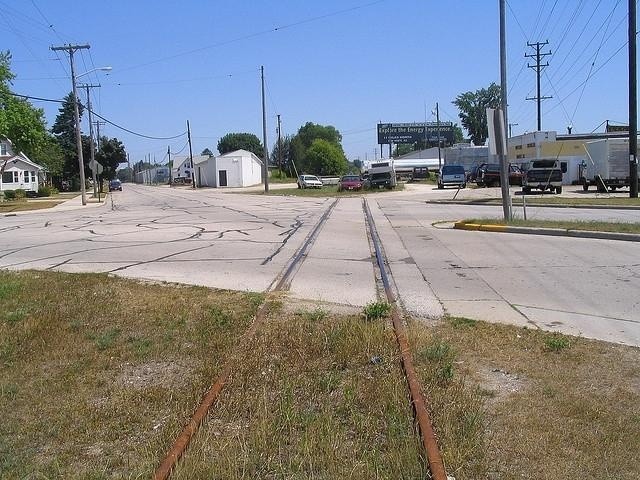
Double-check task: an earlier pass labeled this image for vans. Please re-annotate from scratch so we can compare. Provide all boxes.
[520,157,563,193]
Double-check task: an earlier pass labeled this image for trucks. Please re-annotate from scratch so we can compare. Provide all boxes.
[581,134,640,193]
[359,158,444,180]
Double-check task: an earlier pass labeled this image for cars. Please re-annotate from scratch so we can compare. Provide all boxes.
[295,174,324,190]
[109,178,123,192]
[335,174,362,193]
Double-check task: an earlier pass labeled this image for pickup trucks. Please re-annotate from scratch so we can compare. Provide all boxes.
[412,166,430,182]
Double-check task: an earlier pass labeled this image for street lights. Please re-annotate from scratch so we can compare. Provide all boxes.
[73,67,112,206]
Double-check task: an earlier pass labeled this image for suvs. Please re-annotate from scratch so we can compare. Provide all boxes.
[475,161,524,188]
[371,172,394,190]
[436,163,467,190]
